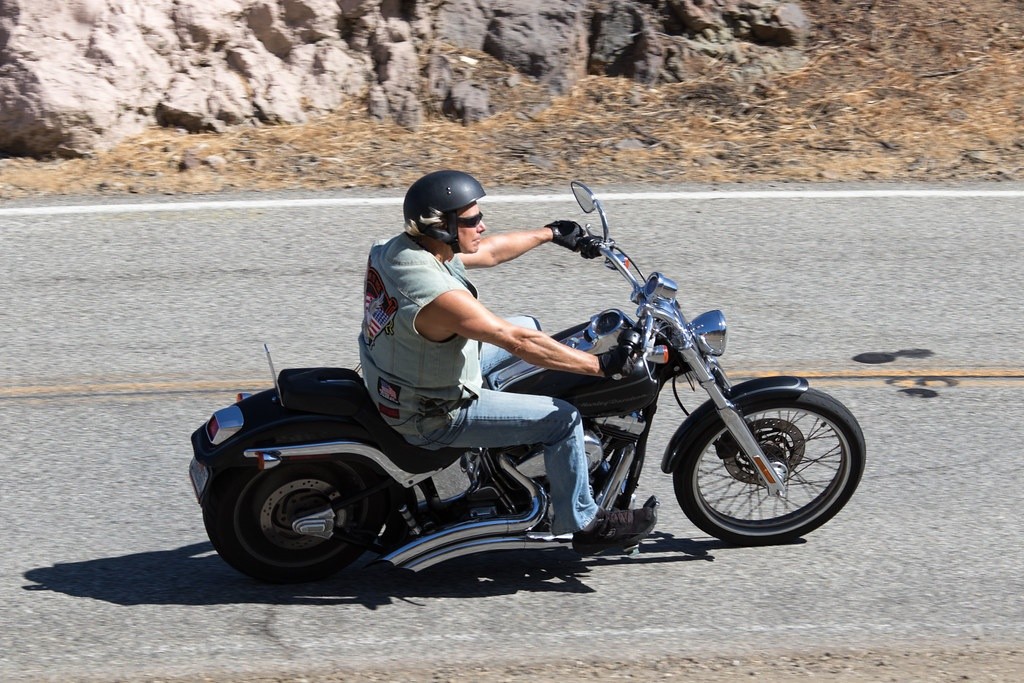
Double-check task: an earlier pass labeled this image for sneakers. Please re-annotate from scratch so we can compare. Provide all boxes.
[572,507,657,556]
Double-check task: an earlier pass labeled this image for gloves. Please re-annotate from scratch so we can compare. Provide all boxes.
[600,348,637,379]
[545,219,585,253]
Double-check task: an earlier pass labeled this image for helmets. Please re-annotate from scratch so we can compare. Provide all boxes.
[404,170,485,226]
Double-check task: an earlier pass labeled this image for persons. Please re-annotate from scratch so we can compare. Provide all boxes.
[359,170,658,555]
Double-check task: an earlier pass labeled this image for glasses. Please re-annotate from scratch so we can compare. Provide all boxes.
[455,212,483,228]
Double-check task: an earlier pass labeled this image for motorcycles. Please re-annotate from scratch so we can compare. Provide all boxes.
[188,177,870,587]
